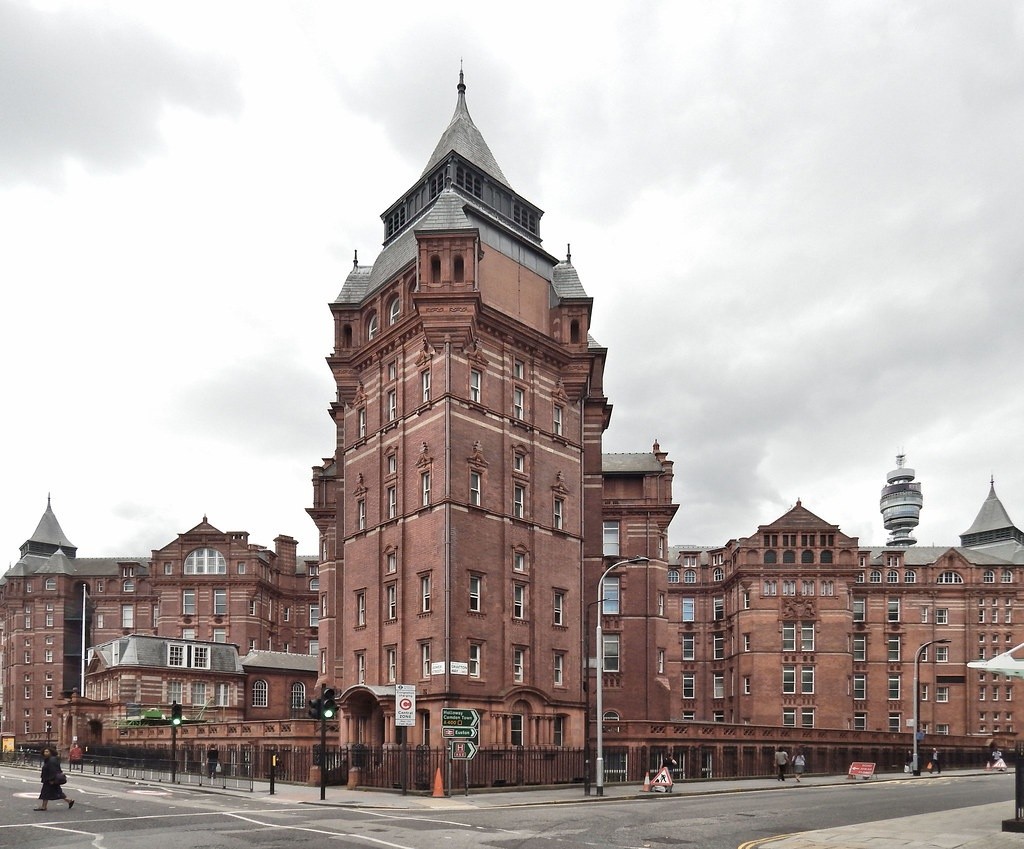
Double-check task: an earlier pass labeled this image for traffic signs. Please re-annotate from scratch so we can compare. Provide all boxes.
[441,708,480,760]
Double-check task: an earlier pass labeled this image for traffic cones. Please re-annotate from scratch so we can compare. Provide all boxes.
[639,772,651,792]
[984,761,992,771]
[430,769,451,799]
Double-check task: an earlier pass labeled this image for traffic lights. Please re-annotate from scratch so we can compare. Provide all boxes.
[172,704,183,727]
[308,698,322,719]
[323,688,337,719]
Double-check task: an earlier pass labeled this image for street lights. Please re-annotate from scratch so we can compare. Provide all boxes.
[594,558,652,795]
[583,598,611,796]
[912,639,952,776]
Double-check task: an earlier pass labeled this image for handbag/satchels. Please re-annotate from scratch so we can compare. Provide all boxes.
[927,762,932,769]
[672,759,677,765]
[791,755,797,767]
[53,770,67,785]
[904,765,909,772]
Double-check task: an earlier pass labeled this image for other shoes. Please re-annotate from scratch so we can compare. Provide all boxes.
[777,777,780,781]
[797,779,800,782]
[781,778,785,781]
[33,807,47,811]
[69,800,74,808]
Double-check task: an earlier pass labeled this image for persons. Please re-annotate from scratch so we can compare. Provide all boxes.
[662,753,676,793]
[791,748,805,782]
[33,747,75,812]
[992,748,1002,761]
[68,743,82,769]
[929,747,941,774]
[904,749,913,774]
[774,746,790,782]
